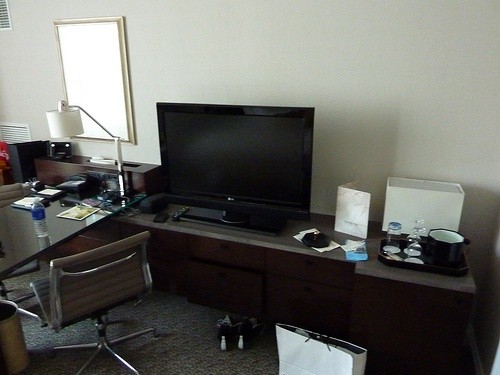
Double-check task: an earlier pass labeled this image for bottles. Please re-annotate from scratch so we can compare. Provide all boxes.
[31,197,49,238]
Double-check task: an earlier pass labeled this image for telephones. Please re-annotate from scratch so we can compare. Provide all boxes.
[55,175,100,195]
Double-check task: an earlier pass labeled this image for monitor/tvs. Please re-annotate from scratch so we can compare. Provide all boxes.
[156,102,315,235]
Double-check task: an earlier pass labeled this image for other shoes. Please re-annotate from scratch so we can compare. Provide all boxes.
[216,314,264,350]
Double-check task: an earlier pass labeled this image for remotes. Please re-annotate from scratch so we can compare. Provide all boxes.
[170,206,190,221]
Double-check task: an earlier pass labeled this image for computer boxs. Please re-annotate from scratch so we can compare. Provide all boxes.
[6,140,51,183]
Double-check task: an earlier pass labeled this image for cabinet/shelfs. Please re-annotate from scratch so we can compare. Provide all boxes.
[31,153,478,375]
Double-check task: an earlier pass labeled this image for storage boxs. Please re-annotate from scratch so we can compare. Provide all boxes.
[382,176,464,237]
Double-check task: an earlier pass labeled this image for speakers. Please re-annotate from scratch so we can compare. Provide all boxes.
[51,142,72,159]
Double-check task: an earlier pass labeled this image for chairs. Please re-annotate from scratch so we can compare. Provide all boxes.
[0,183,48,328]
[31,230,161,375]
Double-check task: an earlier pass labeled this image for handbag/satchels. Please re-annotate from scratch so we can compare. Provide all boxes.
[274,322,368,375]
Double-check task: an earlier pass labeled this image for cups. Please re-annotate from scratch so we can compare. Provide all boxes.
[387,221,402,253]
[428,228,465,267]
[354,239,367,254]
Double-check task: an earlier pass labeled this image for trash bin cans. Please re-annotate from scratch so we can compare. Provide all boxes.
[0,300,31,375]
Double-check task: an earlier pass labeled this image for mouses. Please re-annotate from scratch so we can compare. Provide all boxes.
[154,212,168,222]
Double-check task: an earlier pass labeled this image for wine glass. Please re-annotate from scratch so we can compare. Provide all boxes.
[406,217,428,257]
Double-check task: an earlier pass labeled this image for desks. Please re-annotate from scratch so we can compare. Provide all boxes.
[0,187,139,276]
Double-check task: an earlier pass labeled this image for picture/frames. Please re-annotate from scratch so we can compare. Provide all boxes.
[53,16,137,146]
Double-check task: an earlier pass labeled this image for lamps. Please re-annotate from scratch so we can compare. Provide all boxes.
[46,100,128,197]
